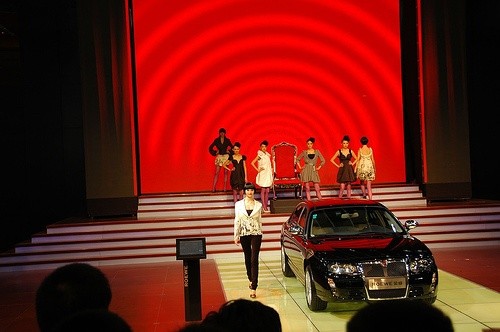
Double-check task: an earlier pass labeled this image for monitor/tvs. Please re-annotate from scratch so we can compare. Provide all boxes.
[176,238,206,260]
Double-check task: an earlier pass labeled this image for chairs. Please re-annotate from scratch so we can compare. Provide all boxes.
[272,142,303,201]
[314,210,372,233]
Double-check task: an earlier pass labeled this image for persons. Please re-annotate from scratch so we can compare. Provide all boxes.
[347,300,456,332]
[330,136,358,199]
[233,183,263,298]
[33,263,113,332]
[208,128,233,193]
[68,308,133,332]
[223,142,247,207]
[354,137,376,200]
[203,299,282,332]
[295,136,326,201]
[251,140,274,211]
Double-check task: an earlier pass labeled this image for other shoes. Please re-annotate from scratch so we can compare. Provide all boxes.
[249,284,252,289]
[250,294,256,298]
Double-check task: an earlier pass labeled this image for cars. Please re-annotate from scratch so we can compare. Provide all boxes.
[281,197,439,312]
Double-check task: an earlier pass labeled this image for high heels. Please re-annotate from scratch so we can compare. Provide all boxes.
[262,208,271,213]
